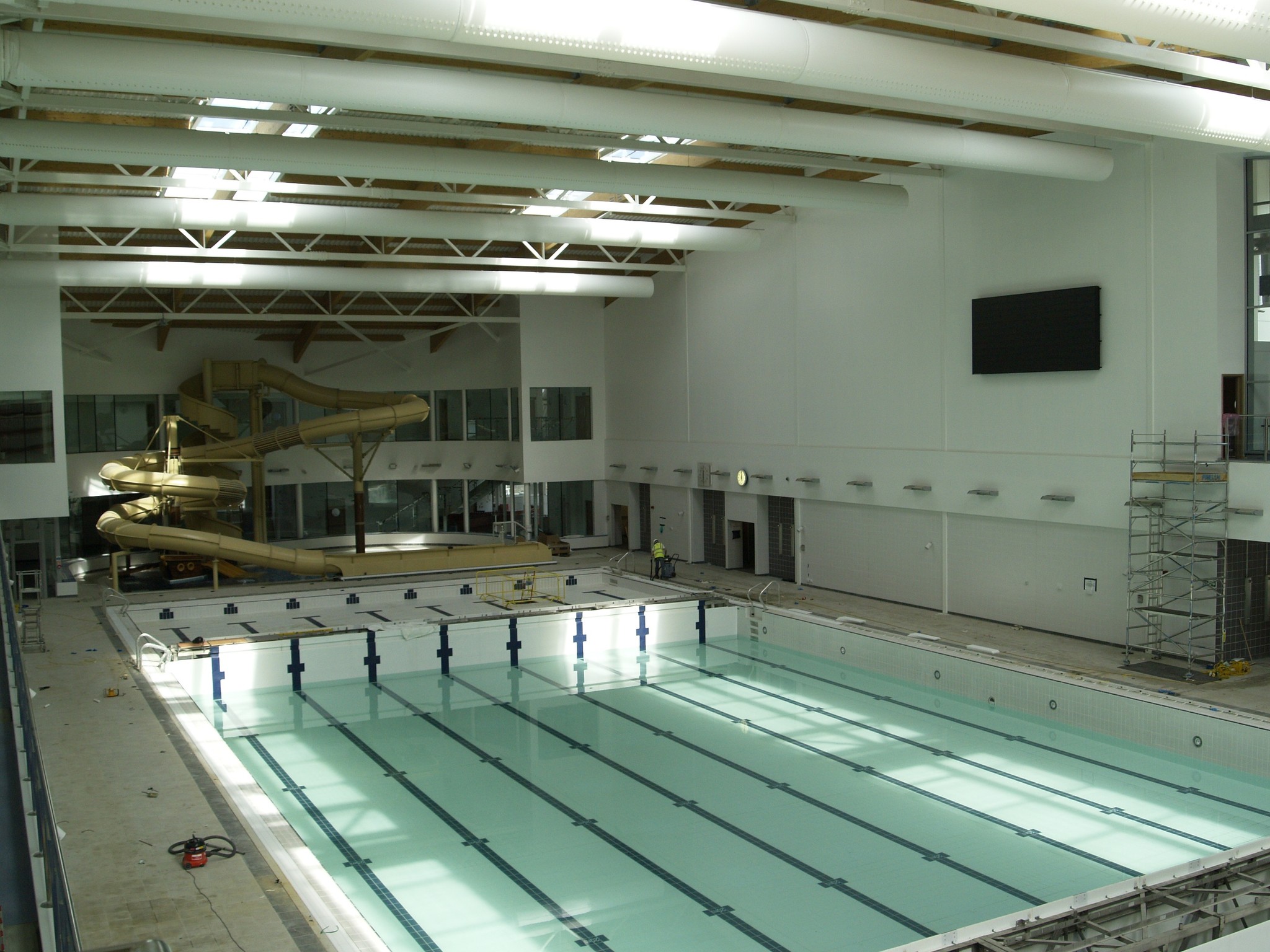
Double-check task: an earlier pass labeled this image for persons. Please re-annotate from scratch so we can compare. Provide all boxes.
[652,539,667,580]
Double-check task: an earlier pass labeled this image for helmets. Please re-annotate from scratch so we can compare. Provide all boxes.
[653,539,658,544]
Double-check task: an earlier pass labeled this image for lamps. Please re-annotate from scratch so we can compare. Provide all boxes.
[847,481,872,488]
[609,463,626,468]
[750,473,773,480]
[389,463,398,469]
[794,477,819,484]
[510,189,594,216]
[598,134,697,164]
[1124,501,1163,508]
[639,466,657,471]
[463,463,471,468]
[967,490,999,497]
[1041,495,1075,502]
[158,97,274,199]
[710,470,730,477]
[233,108,341,201]
[903,485,931,491]
[672,468,692,473]
[1222,508,1264,516]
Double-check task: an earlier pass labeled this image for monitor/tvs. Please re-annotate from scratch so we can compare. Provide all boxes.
[972,285,1101,375]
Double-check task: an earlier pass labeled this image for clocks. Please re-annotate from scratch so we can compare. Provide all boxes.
[737,470,747,486]
